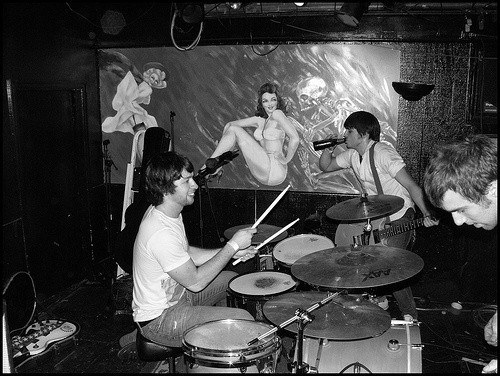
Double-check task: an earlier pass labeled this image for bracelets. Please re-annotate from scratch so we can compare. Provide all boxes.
[227,240,239,252]
[325,148,333,154]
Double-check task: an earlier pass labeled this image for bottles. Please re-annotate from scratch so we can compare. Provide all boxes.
[313,138,346,151]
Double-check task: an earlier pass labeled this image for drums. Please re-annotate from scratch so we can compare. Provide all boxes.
[182,318,281,373]
[226,269,296,326]
[273,234,335,273]
[292,319,424,373]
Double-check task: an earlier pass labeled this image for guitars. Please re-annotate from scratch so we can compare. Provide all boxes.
[11,320,76,358]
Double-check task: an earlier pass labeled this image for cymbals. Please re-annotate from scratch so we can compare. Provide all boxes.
[291,245,424,289]
[224,224,288,243]
[263,291,391,340]
[326,195,404,220]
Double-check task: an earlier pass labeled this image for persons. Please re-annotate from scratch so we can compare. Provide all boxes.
[132,152,258,347]
[319,112,439,320]
[425,134,497,373]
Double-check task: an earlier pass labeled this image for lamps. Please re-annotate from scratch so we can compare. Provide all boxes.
[335,2,372,28]
[170,2,203,34]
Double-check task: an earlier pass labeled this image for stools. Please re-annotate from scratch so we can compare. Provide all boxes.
[135,328,180,373]
